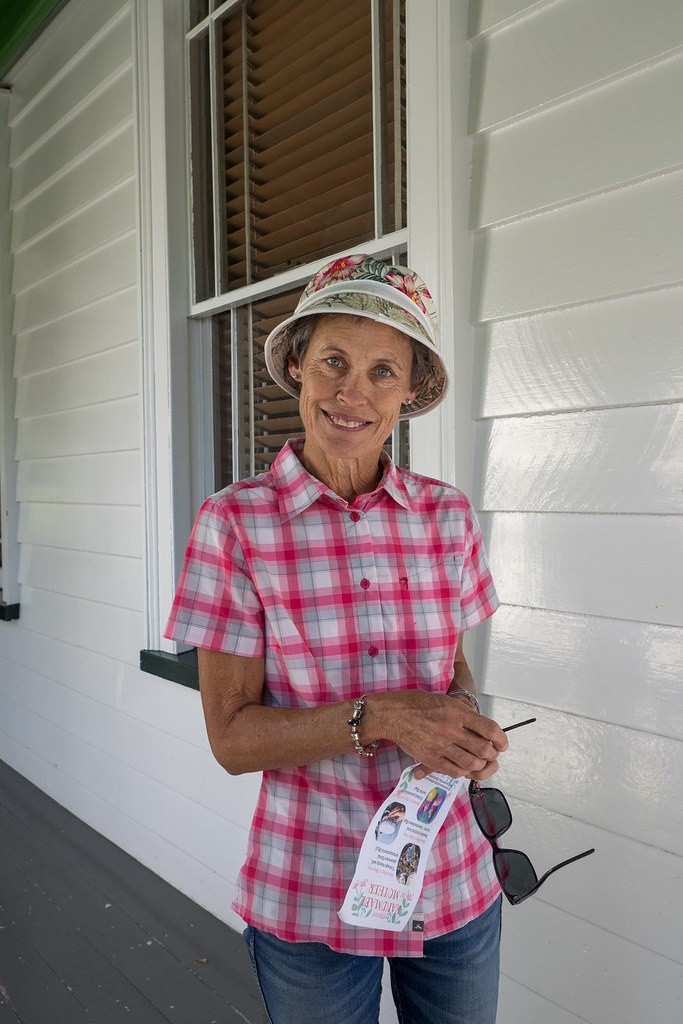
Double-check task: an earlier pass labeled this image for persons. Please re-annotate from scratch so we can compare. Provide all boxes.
[161,256,507,1024]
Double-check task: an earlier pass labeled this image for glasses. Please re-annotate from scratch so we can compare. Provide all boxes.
[465,718,596,907]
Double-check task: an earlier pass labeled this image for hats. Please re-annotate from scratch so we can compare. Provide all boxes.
[263,253,449,424]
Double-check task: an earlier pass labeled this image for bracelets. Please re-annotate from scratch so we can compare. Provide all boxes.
[447,689,482,716]
[346,693,379,758]
